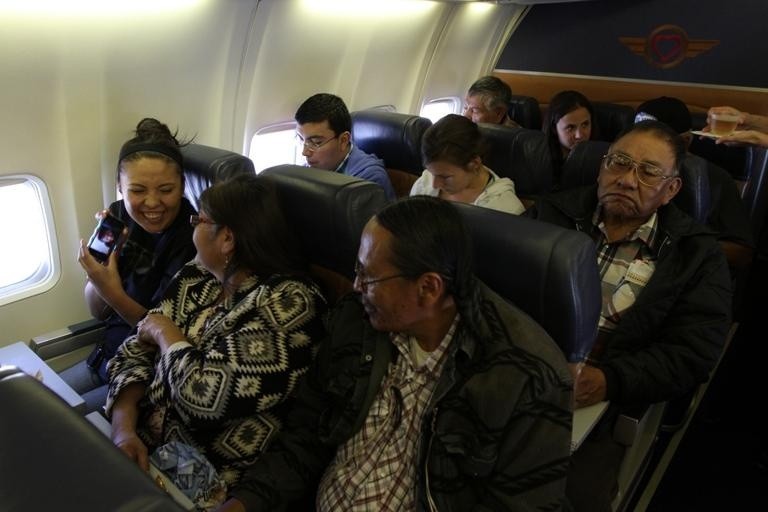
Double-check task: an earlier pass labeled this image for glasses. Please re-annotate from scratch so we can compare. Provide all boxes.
[355,256,453,293]
[190,215,216,227]
[295,134,335,152]
[601,151,681,187]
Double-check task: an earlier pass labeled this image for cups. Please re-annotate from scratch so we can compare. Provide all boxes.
[710,107,740,135]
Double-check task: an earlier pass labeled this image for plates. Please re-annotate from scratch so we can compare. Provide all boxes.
[690,128,741,140]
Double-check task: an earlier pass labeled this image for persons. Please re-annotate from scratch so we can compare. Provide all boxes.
[513,121,735,510]
[460,74,523,132]
[103,173,329,510]
[542,89,599,188]
[635,96,767,295]
[203,194,576,510]
[55,115,201,418]
[294,92,399,209]
[406,113,528,215]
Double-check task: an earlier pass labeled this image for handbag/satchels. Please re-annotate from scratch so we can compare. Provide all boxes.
[142,440,228,511]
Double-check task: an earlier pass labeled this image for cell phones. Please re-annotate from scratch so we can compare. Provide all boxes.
[86,214,124,260]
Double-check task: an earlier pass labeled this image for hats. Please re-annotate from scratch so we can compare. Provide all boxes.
[634,96,691,134]
